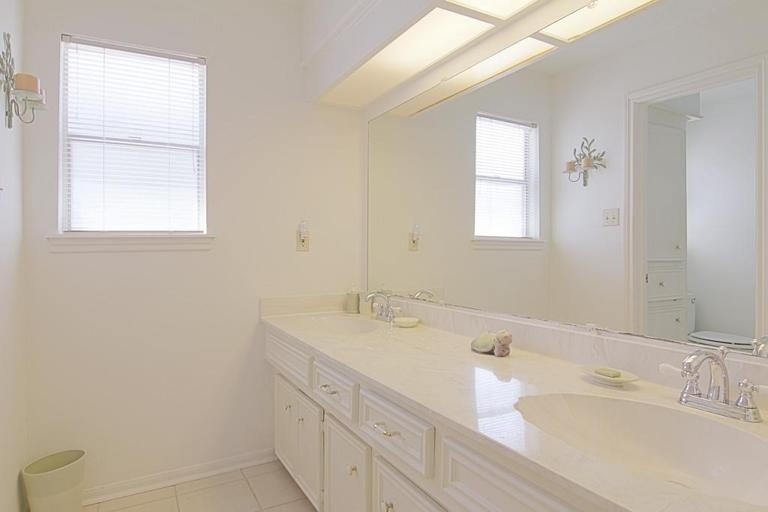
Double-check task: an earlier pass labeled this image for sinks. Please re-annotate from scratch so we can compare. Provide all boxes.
[514,392,768,508]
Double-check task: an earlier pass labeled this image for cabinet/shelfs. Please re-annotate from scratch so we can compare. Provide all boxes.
[373,455,450,512]
[432,429,581,512]
[642,89,704,345]
[263,331,313,392]
[306,351,361,431]
[319,407,373,512]
[355,380,435,482]
[270,369,322,512]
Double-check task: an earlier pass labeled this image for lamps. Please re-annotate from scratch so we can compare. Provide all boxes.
[0,27,50,130]
[553,130,608,189]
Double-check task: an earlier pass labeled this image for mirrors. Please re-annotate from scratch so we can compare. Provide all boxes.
[365,0,762,352]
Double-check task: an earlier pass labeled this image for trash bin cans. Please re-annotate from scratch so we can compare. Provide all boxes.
[22,449,87,512]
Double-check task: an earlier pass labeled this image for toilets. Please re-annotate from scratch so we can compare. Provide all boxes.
[689,330,753,353]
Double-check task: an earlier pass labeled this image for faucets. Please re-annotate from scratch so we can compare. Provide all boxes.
[364,291,390,317]
[680,350,730,404]
[413,289,435,300]
[752,336,768,357]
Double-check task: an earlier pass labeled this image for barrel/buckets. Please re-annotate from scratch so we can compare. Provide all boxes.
[21,447,88,511]
